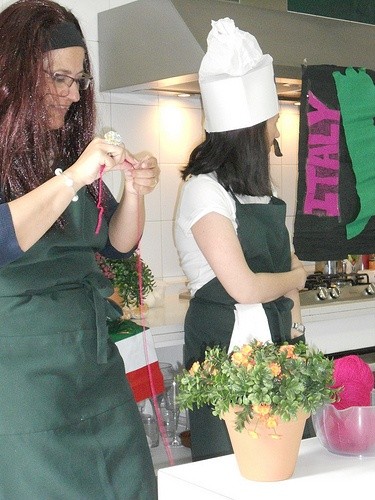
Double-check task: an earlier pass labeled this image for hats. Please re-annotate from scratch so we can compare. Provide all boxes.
[198,18,280,133]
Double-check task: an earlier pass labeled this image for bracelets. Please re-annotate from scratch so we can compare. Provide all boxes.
[291,322,305,332]
[55,168,79,202]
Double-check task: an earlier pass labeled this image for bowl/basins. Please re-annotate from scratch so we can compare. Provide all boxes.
[312,388,375,457]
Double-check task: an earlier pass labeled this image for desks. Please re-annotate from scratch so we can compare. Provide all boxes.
[157,436,375,500]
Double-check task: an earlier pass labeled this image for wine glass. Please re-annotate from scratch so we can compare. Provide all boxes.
[149,363,190,448]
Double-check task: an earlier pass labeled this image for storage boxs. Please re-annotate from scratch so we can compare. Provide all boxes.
[109,320,167,403]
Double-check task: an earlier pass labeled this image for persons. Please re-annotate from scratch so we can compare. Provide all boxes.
[173,17,316,462]
[0,0,160,500]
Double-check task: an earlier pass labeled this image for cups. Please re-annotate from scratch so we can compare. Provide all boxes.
[141,414,159,447]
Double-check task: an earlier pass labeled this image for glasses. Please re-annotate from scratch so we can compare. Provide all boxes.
[43,69,93,90]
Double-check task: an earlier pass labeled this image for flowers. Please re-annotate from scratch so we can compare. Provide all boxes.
[172,338,344,438]
[95,252,157,309]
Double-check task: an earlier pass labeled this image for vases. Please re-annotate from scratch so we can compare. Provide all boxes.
[220,403,310,482]
[107,288,125,308]
